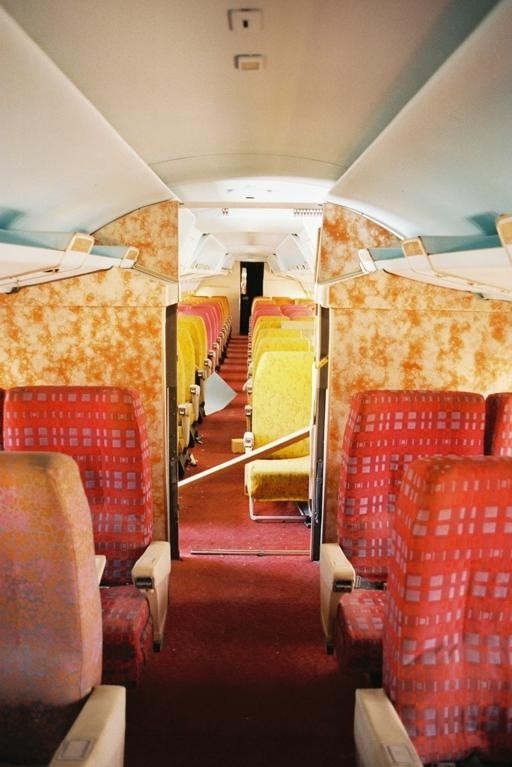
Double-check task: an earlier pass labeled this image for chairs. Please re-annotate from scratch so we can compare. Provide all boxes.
[483,387,510,458]
[354,451,512,766]
[243,296,316,521]
[2,383,174,676]
[2,449,126,766]
[319,389,486,670]
[177,295,233,479]
